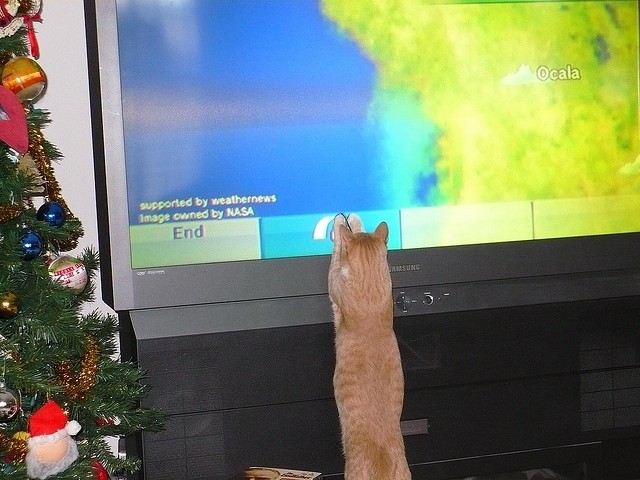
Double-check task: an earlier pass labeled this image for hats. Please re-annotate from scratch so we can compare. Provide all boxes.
[28,401,82,444]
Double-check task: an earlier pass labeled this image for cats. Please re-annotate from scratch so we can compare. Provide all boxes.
[327,213,412,480]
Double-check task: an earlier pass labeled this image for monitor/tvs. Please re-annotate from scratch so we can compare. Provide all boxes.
[81,1,636,418]
